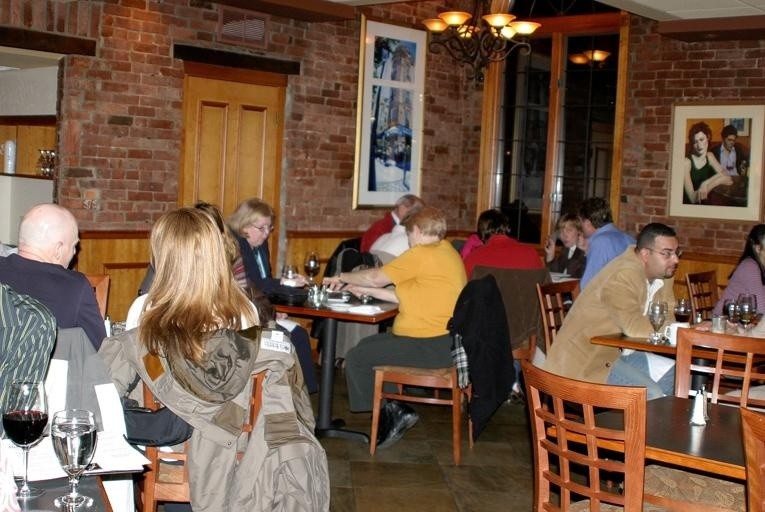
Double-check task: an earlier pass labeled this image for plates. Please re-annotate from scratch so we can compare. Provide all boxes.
[328,299,345,303]
[328,304,350,308]
[342,293,351,302]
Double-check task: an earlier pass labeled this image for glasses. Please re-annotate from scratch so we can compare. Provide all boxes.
[641,247,683,257]
[250,223,274,233]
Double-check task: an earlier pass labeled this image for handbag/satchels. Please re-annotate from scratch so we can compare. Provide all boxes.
[121,373,194,448]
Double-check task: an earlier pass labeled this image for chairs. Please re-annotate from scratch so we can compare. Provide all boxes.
[740,407,765,512]
[370,274,516,463]
[536,279,616,491]
[137,326,330,511]
[520,358,684,511]
[620,327,765,511]
[80,273,110,320]
[471,265,546,363]
[685,270,719,325]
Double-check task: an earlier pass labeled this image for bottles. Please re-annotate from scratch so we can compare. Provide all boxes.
[361,295,373,304]
[697,314,703,323]
[35,149,44,175]
[691,389,706,425]
[43,150,50,176]
[700,384,711,420]
[308,284,326,310]
[104,316,112,337]
[49,150,55,176]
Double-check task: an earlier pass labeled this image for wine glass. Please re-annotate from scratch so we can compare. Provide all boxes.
[2,381,48,500]
[739,302,753,337]
[304,252,320,286]
[52,410,97,510]
[648,301,665,346]
[726,303,739,336]
[674,298,691,322]
[723,299,737,316]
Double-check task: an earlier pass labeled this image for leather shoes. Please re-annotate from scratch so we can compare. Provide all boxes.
[376,400,420,450]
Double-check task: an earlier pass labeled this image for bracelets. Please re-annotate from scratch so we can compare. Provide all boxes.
[336,272,344,288]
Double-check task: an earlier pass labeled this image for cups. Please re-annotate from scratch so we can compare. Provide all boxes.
[282,265,298,280]
[711,318,726,334]
[665,323,690,346]
[111,321,127,336]
[736,294,757,318]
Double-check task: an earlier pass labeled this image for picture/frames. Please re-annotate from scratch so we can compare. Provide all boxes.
[509,47,569,213]
[665,102,765,224]
[351,13,429,210]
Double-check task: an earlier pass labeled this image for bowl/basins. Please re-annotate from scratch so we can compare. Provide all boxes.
[328,292,341,299]
[265,286,308,307]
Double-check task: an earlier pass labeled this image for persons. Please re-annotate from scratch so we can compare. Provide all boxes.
[369,206,419,267]
[575,198,637,292]
[462,231,484,256]
[228,201,318,395]
[710,125,744,177]
[684,122,734,204]
[543,224,693,401]
[322,207,468,447]
[465,209,543,404]
[544,214,586,278]
[126,208,259,465]
[1,203,108,352]
[196,203,248,295]
[713,224,765,319]
[360,195,425,256]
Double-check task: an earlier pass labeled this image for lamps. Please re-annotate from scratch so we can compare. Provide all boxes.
[420,1,542,81]
[568,50,612,69]
